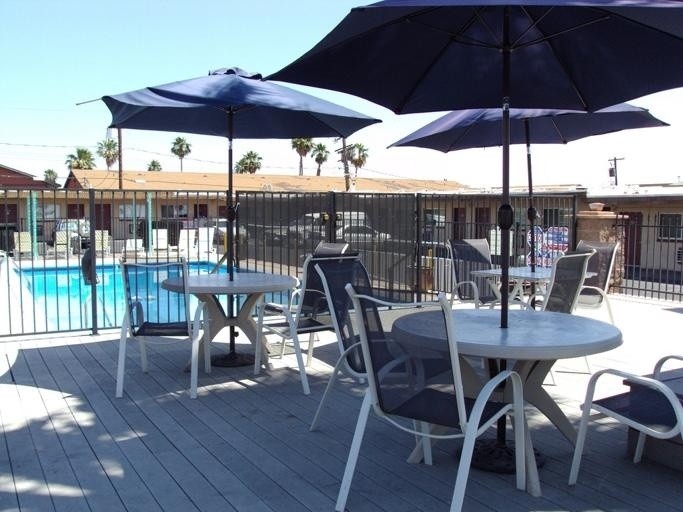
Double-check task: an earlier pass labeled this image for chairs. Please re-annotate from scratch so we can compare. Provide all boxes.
[260,240,350,360]
[307,257,453,468]
[254,254,358,396]
[6,221,218,264]
[447,238,621,387]
[568,354,682,486]
[333,282,526,512]
[114,257,212,399]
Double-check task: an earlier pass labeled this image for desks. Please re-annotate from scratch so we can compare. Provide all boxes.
[470,266,599,309]
[160,273,302,376]
[390,308,625,498]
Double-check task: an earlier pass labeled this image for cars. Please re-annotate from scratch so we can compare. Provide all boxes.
[264,215,303,246]
[320,224,390,243]
[204,219,250,244]
[51,218,91,247]
[136,219,166,241]
[169,219,200,242]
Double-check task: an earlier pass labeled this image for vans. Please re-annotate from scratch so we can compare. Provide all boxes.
[423,208,445,229]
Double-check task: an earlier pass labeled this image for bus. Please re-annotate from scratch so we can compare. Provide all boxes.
[287,211,371,245]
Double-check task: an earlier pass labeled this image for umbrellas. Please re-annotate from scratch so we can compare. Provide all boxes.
[97,64,383,366]
[251,1,683,472]
[385,102,673,307]
